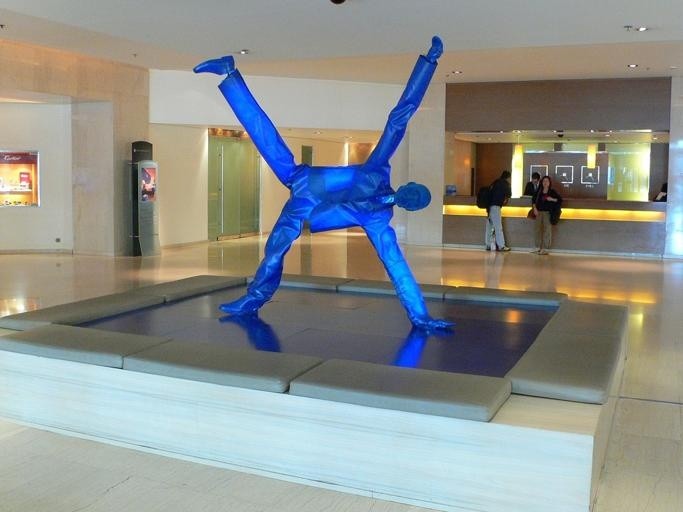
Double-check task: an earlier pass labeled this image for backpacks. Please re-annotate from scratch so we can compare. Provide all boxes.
[477,186,493,208]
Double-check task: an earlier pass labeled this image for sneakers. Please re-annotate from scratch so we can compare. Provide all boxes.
[485,244,511,251]
[530,248,552,255]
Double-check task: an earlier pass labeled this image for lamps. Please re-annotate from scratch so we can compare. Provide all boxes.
[587,144,597,169]
[514,135,523,168]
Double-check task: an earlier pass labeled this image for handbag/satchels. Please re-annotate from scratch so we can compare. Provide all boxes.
[550,206,561,225]
[527,209,537,221]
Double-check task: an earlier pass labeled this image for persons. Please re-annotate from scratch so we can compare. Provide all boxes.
[216,313,428,369]
[531,175,562,256]
[191,33,457,333]
[484,170,511,251]
[653,183,666,202]
[523,172,539,195]
